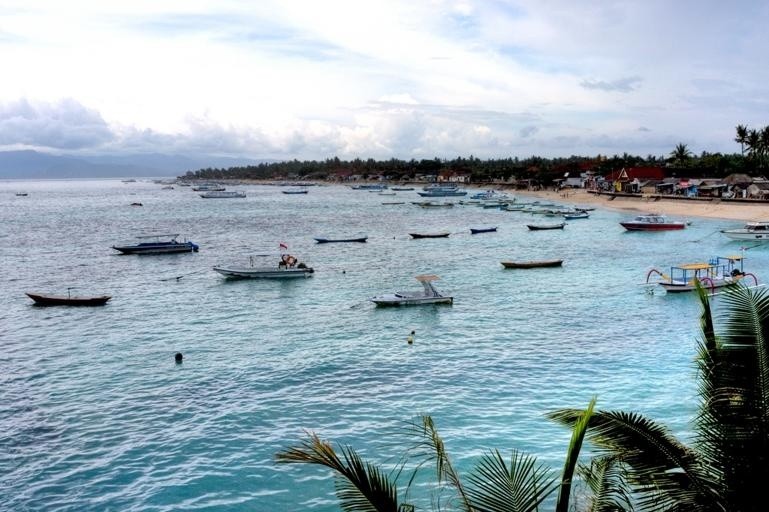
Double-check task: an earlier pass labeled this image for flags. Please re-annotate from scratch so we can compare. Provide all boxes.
[280,243,288,250]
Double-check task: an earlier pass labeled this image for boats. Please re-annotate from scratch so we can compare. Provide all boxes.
[367,275,453,306]
[470,226,497,233]
[502,259,563,269]
[720,222,769,240]
[113,234,199,254]
[313,236,367,242]
[527,222,568,230]
[25,293,112,305]
[636,255,766,297]
[351,184,597,219]
[213,266,316,279]
[408,233,450,238]
[619,216,691,231]
[162,183,314,198]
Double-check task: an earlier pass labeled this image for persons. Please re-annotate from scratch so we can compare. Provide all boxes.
[278,254,290,269]
[286,254,298,268]
